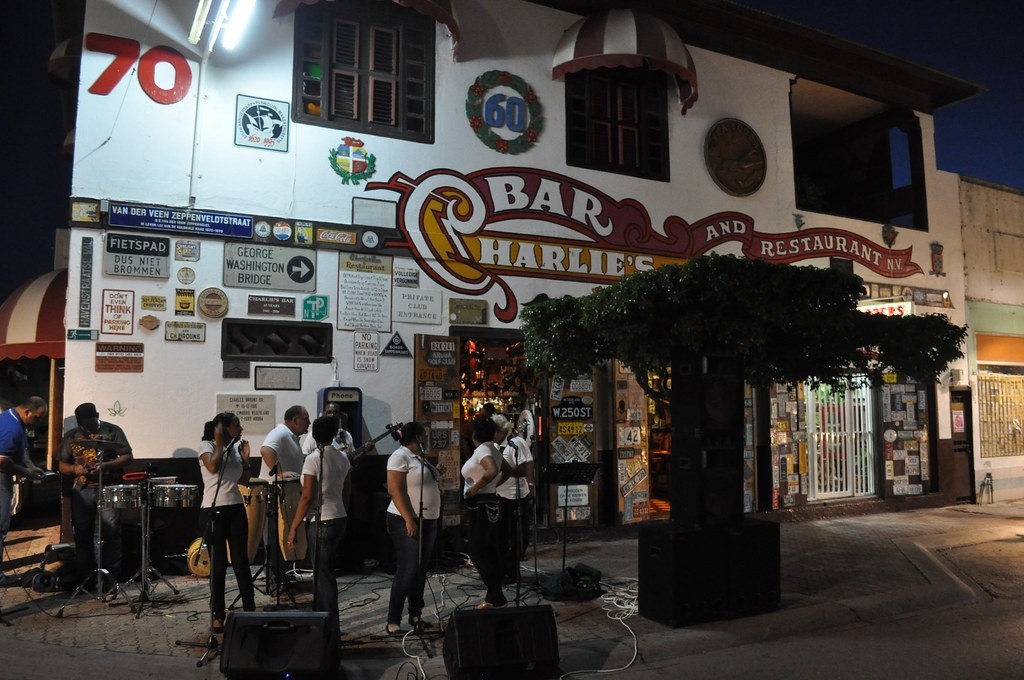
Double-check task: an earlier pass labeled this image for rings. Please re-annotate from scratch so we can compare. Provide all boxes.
[288,542,290,543]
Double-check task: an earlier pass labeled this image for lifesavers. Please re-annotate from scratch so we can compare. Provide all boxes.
[517,409,535,448]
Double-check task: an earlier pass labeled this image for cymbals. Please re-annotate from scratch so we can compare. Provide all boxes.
[70,438,125,450]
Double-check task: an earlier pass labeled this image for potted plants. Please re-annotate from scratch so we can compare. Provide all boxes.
[521,256,962,627]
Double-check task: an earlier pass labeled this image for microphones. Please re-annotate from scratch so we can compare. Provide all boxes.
[223,436,239,453]
[418,441,425,454]
[317,442,324,452]
[269,461,281,476]
[508,441,519,450]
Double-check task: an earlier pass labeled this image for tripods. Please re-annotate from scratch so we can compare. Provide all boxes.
[174,450,233,668]
[510,445,543,601]
[229,468,300,610]
[56,448,180,620]
[369,453,446,659]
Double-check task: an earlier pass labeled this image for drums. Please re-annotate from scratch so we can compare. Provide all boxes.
[152,483,200,508]
[102,483,143,509]
[187,537,212,577]
[226,477,271,564]
[273,476,310,561]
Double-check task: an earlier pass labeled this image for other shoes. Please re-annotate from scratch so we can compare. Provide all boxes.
[475,600,508,609]
[507,582,517,588]
[212,625,224,634]
[386,624,405,636]
[0,575,16,586]
[408,617,429,628]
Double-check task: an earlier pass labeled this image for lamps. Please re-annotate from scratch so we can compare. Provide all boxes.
[188,0,258,51]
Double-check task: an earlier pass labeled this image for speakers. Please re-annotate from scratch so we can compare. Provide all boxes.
[220,611,342,680]
[442,603,560,680]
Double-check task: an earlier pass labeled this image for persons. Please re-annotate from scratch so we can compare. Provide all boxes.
[287,404,376,611]
[198,414,257,631]
[57,403,133,594]
[460,400,536,607]
[260,405,311,598]
[0,397,48,586]
[385,422,447,639]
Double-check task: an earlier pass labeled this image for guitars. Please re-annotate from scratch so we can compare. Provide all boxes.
[348,421,404,461]
[512,419,529,437]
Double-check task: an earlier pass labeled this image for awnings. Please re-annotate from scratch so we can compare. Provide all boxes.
[272,0,461,60]
[553,8,698,109]
[0,269,68,358]
